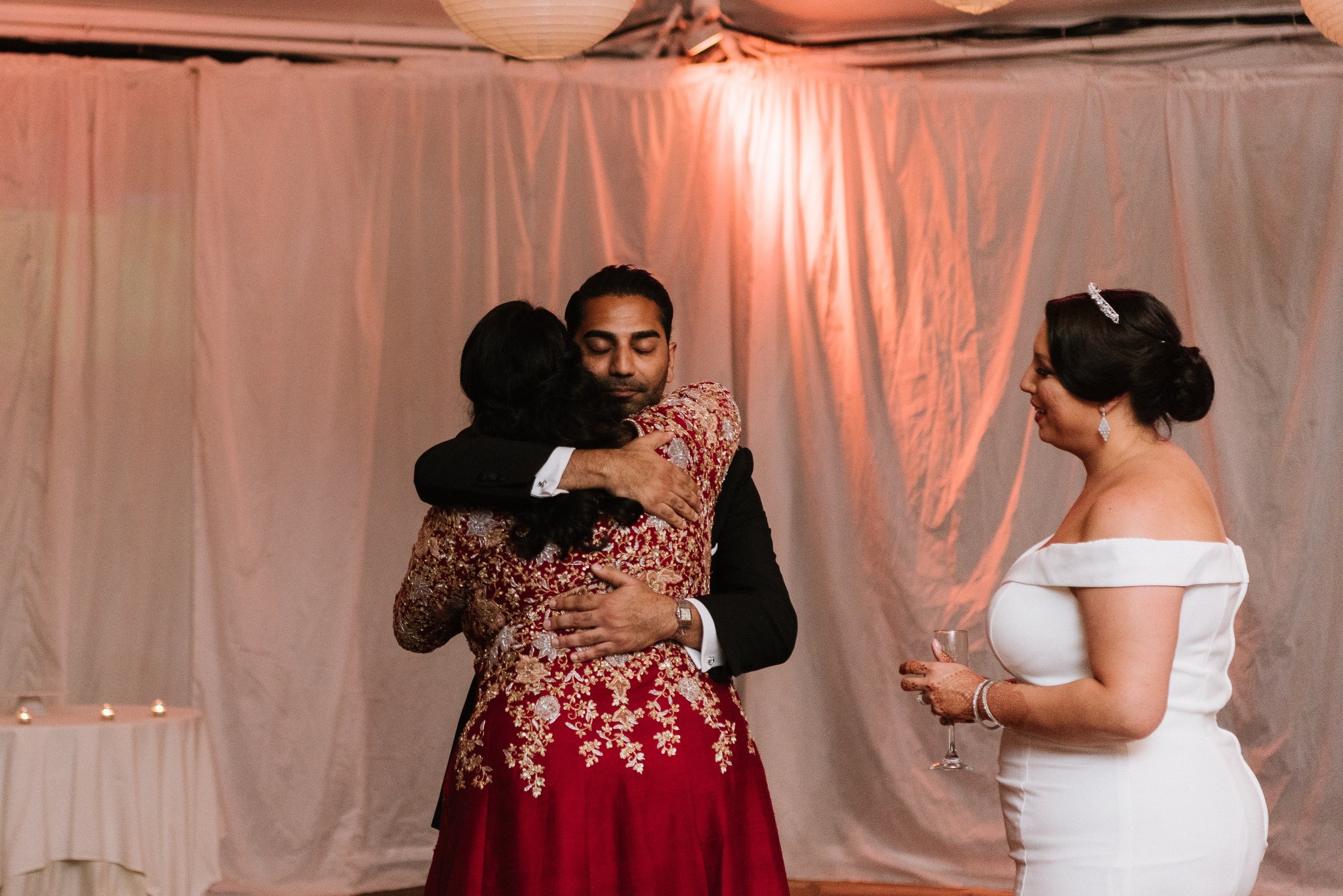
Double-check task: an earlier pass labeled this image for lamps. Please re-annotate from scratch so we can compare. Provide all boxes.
[682,0,725,55]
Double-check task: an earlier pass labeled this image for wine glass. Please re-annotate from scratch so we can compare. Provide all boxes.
[928,630,972,771]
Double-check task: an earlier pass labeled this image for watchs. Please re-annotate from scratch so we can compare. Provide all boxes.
[670,600,691,641]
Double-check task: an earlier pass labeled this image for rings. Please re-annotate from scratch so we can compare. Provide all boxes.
[917,692,927,705]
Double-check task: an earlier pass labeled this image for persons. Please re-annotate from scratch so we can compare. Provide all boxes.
[390,297,790,895]
[899,280,1269,896]
[410,261,797,832]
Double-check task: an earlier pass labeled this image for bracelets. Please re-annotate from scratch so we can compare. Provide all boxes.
[973,679,1002,732]
[981,679,1011,730]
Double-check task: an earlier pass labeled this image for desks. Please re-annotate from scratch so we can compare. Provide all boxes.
[1,703,232,896]
